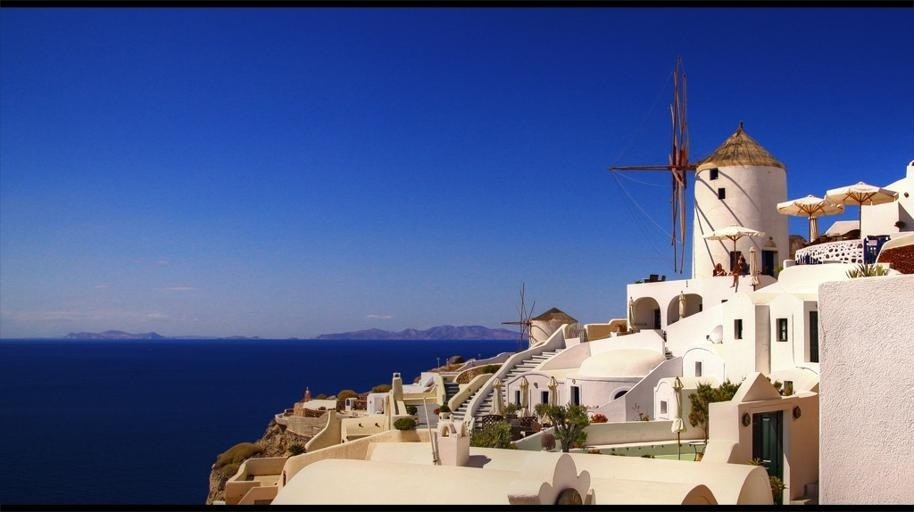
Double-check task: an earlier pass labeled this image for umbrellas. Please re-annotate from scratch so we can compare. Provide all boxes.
[677,290,687,321]
[491,376,506,415]
[823,181,900,237]
[541,376,560,426]
[627,297,638,334]
[775,194,845,242]
[700,223,768,263]
[809,216,818,242]
[748,246,760,291]
[517,376,531,418]
[670,376,687,460]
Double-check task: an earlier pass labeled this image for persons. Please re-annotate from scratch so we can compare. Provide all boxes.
[729,256,746,293]
[712,263,726,276]
